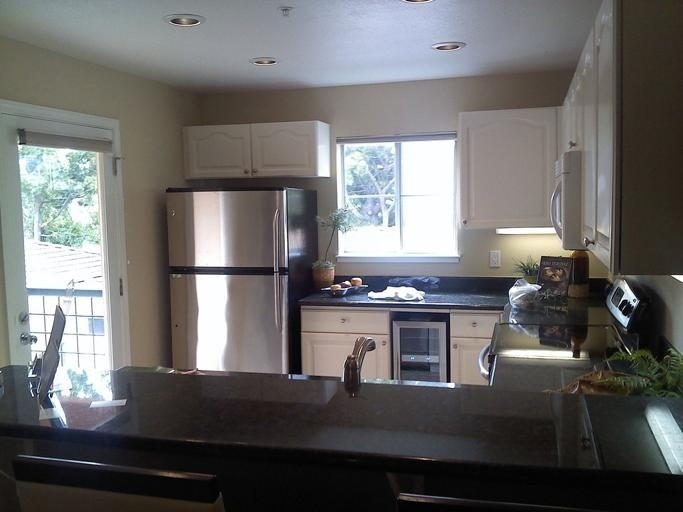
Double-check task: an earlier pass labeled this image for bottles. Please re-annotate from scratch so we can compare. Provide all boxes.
[567,250,589,299]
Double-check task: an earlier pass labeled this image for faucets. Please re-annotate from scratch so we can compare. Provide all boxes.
[345,336,377,386]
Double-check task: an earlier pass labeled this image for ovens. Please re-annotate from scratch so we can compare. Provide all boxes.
[390,314,450,383]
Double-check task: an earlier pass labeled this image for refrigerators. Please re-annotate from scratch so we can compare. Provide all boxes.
[164,184,319,377]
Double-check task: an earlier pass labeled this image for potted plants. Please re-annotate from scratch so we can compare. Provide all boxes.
[311,203,359,290]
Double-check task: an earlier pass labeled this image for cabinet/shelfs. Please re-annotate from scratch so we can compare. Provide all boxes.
[560,1,682,276]
[182,120,330,182]
[300,306,505,387]
[457,105,562,230]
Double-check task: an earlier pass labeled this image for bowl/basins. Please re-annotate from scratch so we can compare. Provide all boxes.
[320,284,369,298]
